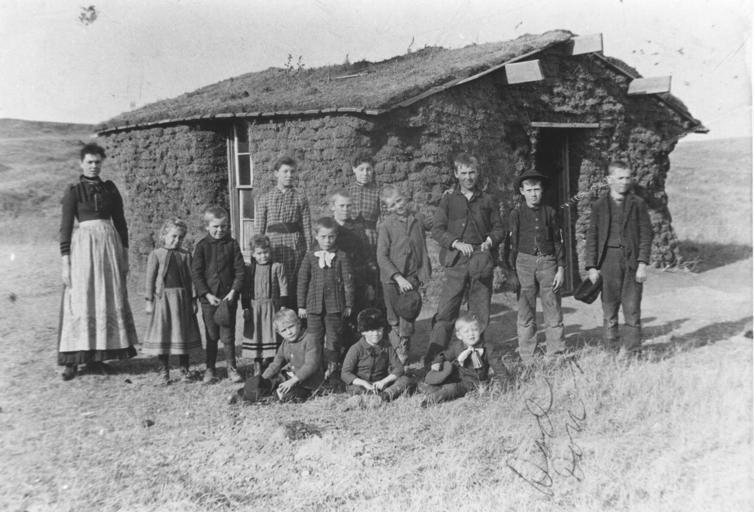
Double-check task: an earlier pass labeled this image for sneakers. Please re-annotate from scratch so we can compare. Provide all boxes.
[340,395,382,411]
[63,366,77,379]
[89,362,110,372]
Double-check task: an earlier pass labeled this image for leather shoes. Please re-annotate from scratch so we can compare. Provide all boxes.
[254,367,262,376]
[180,370,191,382]
[204,368,214,381]
[227,368,240,381]
[160,371,169,385]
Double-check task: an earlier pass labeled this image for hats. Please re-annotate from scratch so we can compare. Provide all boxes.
[512,169,549,194]
[574,274,602,304]
[469,250,494,279]
[358,309,387,329]
[213,299,230,326]
[242,376,272,401]
[394,290,422,320]
[425,361,453,385]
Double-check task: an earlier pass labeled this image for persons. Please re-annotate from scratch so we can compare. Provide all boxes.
[232,308,324,405]
[297,217,354,378]
[586,160,653,359]
[313,191,378,344]
[425,152,504,367]
[256,158,311,311]
[57,145,139,381]
[376,185,434,356]
[421,313,508,409]
[505,169,567,363]
[339,308,418,402]
[142,215,198,383]
[344,159,390,302]
[241,233,288,385]
[193,206,247,383]
